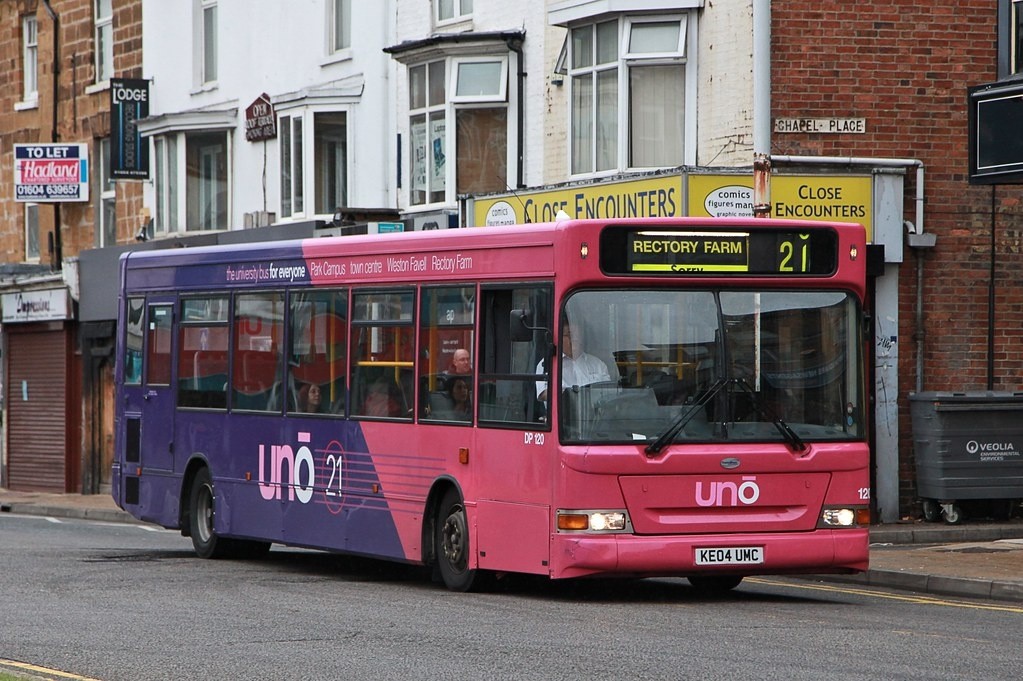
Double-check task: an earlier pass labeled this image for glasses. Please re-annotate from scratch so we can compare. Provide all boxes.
[563,331,585,339]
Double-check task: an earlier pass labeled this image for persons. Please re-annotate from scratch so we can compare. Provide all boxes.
[295,383,322,413]
[439,348,472,391]
[442,377,473,421]
[536,319,611,410]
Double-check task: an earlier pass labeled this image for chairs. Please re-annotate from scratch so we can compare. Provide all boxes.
[183,379,456,420]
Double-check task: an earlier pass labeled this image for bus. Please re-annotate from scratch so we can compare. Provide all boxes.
[111,211,873,593]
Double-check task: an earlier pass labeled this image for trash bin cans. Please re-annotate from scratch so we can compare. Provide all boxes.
[907,390,1023,525]
